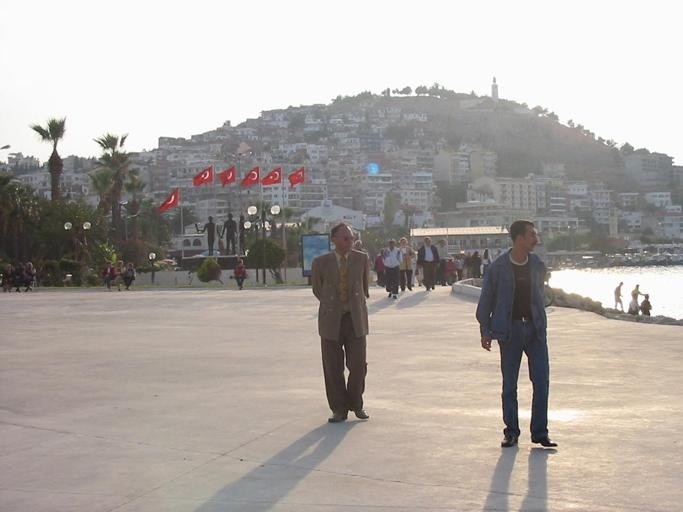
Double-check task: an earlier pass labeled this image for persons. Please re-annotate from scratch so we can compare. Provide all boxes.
[4,262,36,293]
[355,236,502,298]
[101,260,136,293]
[312,222,369,422]
[197,214,246,256]
[235,259,247,290]
[615,282,652,316]
[476,220,558,447]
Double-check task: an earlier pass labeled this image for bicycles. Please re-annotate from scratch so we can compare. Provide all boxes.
[541,262,554,308]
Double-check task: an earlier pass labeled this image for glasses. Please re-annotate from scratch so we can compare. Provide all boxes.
[344,236,355,241]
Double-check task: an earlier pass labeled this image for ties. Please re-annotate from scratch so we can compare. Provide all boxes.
[338,257,347,303]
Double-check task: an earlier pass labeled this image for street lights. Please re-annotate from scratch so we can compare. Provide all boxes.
[243,202,263,285]
[566,225,579,256]
[262,203,284,284]
[62,220,92,262]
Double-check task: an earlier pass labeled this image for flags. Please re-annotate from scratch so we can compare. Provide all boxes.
[220,167,235,187]
[288,168,304,187]
[241,167,259,187]
[155,189,179,215]
[193,166,212,186]
[262,168,281,185]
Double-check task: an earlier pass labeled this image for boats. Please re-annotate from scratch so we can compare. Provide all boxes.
[162,229,206,261]
[581,249,683,266]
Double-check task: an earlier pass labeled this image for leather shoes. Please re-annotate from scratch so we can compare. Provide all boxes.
[501,436,517,447]
[532,438,557,446]
[354,407,368,419]
[329,414,346,422]
